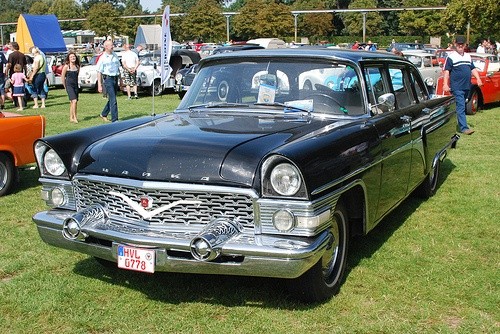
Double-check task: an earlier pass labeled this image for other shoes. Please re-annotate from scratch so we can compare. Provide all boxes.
[128,96,132,99]
[135,94,139,99]
[464,129,474,135]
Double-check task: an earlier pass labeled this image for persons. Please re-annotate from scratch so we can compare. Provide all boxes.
[0,46,8,109]
[351,39,405,57]
[131,42,144,57]
[441,34,484,135]
[6,40,28,106]
[8,62,30,112]
[475,37,500,61]
[95,39,123,123]
[29,47,48,110]
[60,50,82,124]
[119,43,139,100]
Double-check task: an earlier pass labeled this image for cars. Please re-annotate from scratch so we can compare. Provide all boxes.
[44,38,500,102]
[0,110,46,197]
[401,51,443,89]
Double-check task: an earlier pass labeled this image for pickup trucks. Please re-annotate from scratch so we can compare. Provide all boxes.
[32,48,461,298]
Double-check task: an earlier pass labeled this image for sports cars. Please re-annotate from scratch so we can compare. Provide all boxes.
[436,56,500,115]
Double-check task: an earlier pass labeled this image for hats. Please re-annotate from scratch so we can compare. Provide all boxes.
[455,36,466,44]
